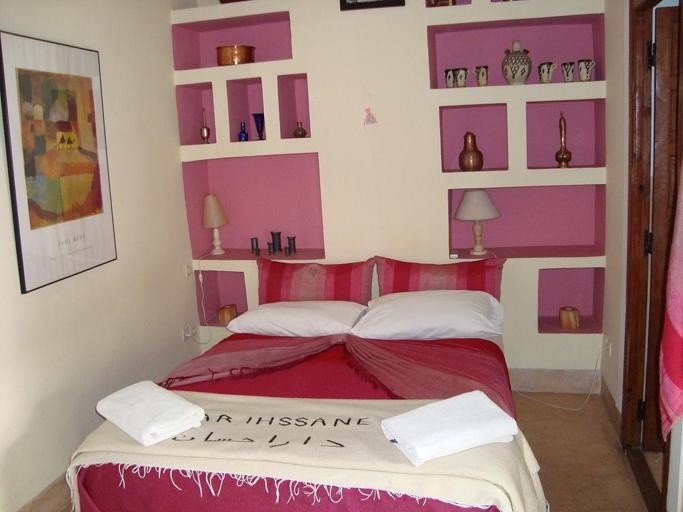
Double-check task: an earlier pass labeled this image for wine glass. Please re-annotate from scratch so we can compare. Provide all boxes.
[251,112,265,140]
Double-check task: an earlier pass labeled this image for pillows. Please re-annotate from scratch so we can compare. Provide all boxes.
[254,254,375,306]
[348,288,505,341]
[224,299,368,338]
[375,255,508,303]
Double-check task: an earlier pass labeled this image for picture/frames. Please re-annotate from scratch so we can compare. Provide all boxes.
[339,0,405,12]
[0,29,119,295]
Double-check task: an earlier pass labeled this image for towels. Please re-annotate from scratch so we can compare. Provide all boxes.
[378,388,520,469]
[94,378,207,448]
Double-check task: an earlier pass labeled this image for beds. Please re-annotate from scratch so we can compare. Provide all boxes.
[64,333,553,512]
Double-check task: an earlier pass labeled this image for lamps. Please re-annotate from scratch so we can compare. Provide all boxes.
[453,189,502,256]
[200,191,230,256]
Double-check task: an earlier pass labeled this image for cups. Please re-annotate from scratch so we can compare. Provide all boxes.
[538,58,596,82]
[442,64,488,87]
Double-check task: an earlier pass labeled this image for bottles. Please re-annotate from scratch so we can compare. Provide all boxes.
[292,121,308,137]
[249,231,296,255]
[554,116,572,168]
[458,133,483,170]
[238,122,249,142]
[502,42,532,85]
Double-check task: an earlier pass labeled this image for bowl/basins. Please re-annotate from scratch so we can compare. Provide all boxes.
[215,45,256,68]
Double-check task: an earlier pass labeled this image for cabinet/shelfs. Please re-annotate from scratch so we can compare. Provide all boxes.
[169,0,606,370]
